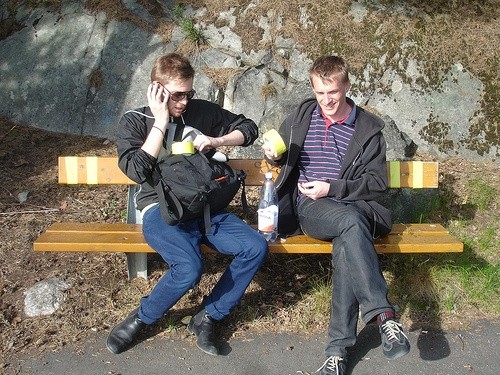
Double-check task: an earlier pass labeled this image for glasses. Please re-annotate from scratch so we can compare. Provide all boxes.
[156,82,196,101]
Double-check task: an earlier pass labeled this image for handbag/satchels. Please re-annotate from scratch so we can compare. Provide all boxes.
[150,149,249,235]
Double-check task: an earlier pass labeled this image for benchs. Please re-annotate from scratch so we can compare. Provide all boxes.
[33,156,463,281]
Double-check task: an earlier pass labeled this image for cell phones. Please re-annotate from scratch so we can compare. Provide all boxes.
[151,84,170,102]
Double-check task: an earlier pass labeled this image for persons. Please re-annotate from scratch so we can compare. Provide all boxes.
[258,54,411,375]
[105,53,269,356]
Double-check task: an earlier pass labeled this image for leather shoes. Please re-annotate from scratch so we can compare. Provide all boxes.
[187,304,221,356]
[105,306,146,355]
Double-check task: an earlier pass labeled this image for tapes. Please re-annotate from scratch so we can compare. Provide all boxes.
[262,128,287,158]
[171,141,195,155]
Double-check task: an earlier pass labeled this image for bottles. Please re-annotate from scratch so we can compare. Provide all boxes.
[257,172,279,243]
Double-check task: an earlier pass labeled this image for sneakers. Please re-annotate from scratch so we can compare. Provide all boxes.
[378,318,411,361]
[313,355,348,375]
